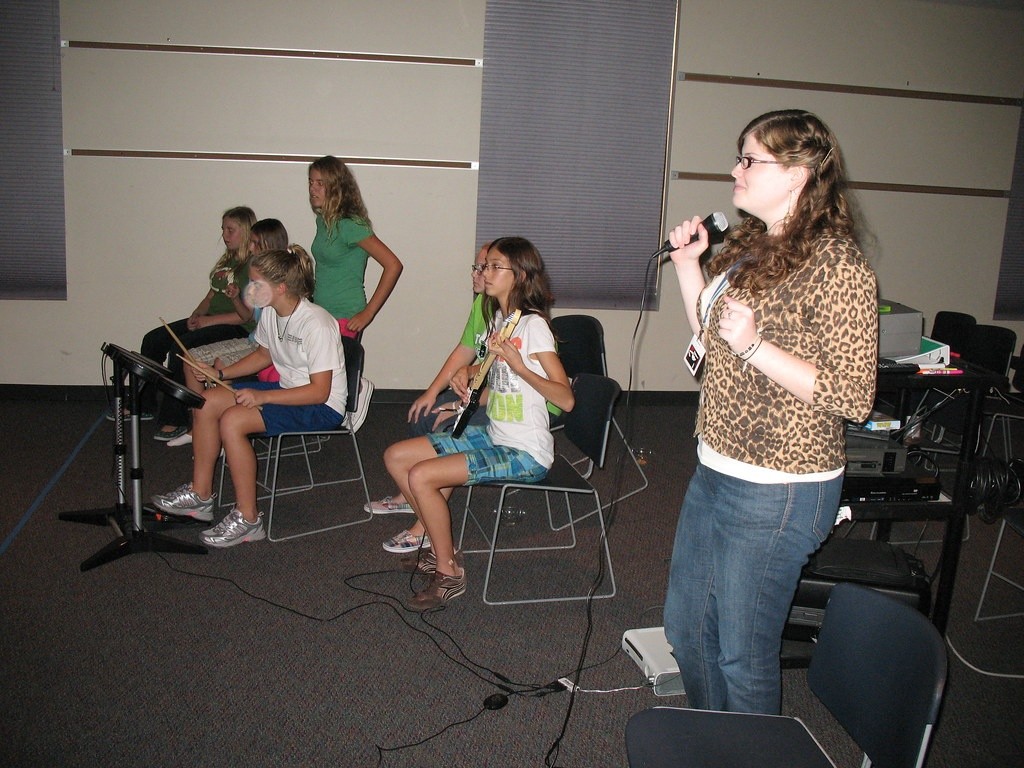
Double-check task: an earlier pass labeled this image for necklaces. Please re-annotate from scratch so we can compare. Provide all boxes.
[276,295,301,342]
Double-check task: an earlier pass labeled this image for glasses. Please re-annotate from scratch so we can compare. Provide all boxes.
[485,264,513,271]
[472,265,486,271]
[736,156,777,170]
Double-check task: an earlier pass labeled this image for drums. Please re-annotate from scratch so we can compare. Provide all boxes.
[100,341,156,369]
[110,348,165,386]
[130,350,173,378]
[157,375,207,410]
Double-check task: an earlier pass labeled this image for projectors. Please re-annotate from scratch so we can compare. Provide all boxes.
[622,627,686,696]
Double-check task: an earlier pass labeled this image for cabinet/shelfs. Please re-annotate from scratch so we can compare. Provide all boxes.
[782,364,988,672]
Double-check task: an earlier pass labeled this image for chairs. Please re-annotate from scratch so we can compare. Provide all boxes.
[624,581,949,768]
[212,425,331,461]
[974,508,1024,622]
[459,373,623,607]
[915,311,1024,473]
[219,335,374,543]
[543,315,649,533]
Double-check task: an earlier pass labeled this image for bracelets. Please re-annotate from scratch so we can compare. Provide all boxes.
[452,400,457,411]
[218,370,223,381]
[727,334,762,363]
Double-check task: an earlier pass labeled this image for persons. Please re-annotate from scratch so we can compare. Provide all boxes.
[364,241,495,553]
[401,237,575,610]
[150,243,348,546]
[167,218,288,461]
[664,107,879,720]
[105,206,257,441]
[257,155,405,435]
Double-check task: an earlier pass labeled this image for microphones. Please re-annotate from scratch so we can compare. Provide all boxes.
[652,212,729,258]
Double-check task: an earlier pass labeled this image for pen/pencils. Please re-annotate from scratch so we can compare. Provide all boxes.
[893,303,901,311]
[917,368,957,374]
[922,370,965,375]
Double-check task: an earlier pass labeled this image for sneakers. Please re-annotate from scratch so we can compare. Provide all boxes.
[408,567,466,609]
[198,508,267,548]
[150,481,217,521]
[400,549,465,574]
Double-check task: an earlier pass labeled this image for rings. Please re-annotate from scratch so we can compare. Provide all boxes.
[227,291,230,294]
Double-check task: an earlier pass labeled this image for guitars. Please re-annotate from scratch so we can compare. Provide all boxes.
[451,308,523,440]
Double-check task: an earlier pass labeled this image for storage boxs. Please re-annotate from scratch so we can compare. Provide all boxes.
[878,298,921,356]
[883,337,952,366]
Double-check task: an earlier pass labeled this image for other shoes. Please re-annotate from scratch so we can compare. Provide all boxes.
[154,425,187,441]
[364,496,415,513]
[106,412,153,422]
[382,530,432,553]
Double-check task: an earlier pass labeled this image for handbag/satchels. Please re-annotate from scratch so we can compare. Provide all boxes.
[811,538,926,584]
